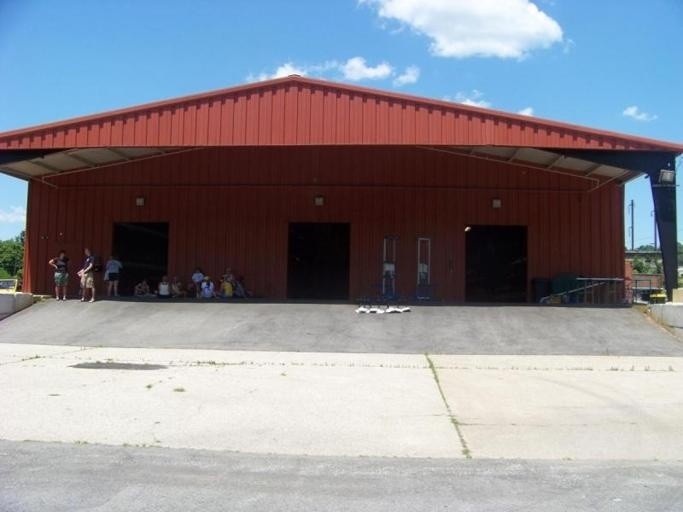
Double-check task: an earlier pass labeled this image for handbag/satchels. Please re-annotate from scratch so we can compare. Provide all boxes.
[104,270,109,281]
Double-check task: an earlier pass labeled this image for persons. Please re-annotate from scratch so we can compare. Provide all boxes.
[48,249,73,301]
[77,247,97,302]
[104,254,124,297]
[134,267,250,300]
